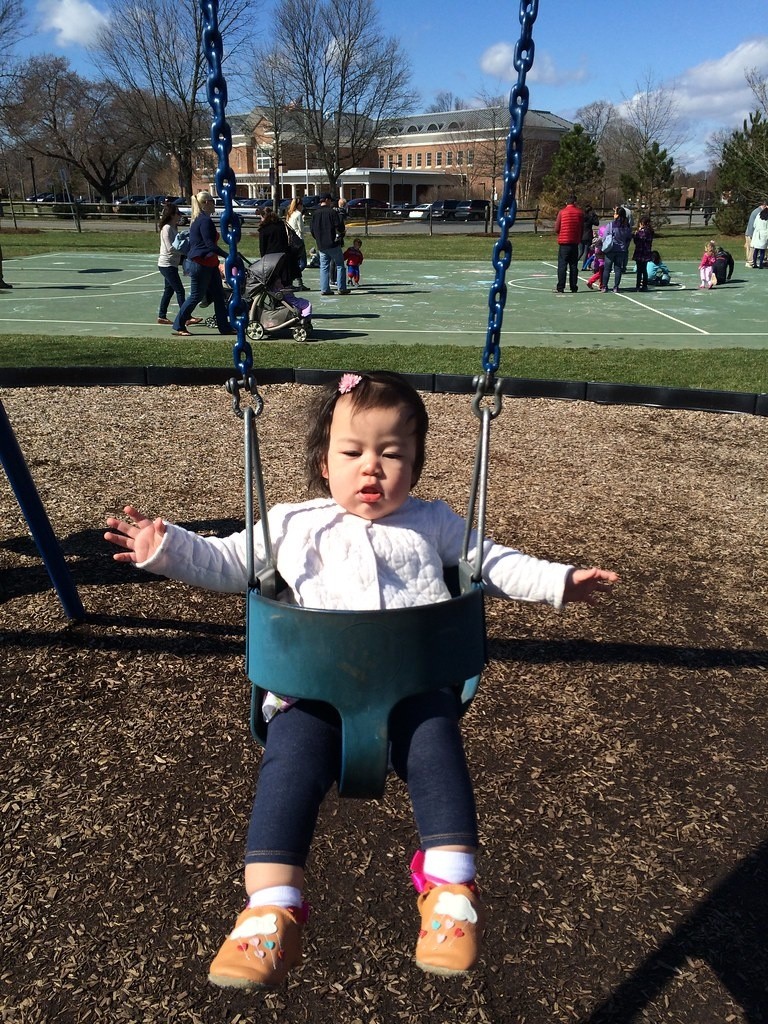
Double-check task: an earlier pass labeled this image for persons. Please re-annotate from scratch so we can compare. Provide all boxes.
[577,205,599,271]
[306,248,320,268]
[555,196,585,293]
[342,238,364,286]
[698,241,735,290]
[309,192,351,295]
[258,196,310,295]
[102,369,617,988]
[582,203,670,293]
[158,203,203,326]
[744,200,768,269]
[172,191,237,336]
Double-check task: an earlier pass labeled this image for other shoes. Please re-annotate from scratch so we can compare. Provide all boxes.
[186,317,204,326]
[572,290,577,292]
[613,287,618,293]
[553,288,564,294]
[745,263,759,268]
[602,287,607,292]
[171,328,191,336]
[321,291,334,295]
[208,900,306,990]
[587,283,594,289]
[349,279,353,286]
[699,285,705,288]
[355,282,359,287]
[709,283,713,289]
[292,284,311,291]
[598,287,602,290]
[635,286,647,292]
[158,317,174,324]
[338,289,351,295]
[416,882,483,976]
[199,302,209,308]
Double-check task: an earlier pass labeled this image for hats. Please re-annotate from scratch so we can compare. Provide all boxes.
[320,193,335,202]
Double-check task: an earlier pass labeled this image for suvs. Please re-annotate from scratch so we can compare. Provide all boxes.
[454,199,498,222]
[428,199,464,221]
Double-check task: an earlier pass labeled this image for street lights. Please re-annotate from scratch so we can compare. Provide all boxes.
[26,157,39,208]
[478,183,485,200]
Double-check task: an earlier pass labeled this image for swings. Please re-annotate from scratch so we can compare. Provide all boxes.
[194,1,542,802]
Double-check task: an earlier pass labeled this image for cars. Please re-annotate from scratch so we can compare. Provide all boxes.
[391,202,419,219]
[26,191,53,202]
[409,203,433,220]
[75,194,329,229]
[35,192,75,207]
[344,197,389,218]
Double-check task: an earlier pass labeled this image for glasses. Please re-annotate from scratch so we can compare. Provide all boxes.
[203,200,216,205]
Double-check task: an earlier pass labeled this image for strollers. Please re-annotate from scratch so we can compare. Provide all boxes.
[220,252,314,343]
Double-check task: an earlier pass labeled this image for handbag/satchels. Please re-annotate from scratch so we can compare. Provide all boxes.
[601,223,614,252]
[281,220,303,251]
